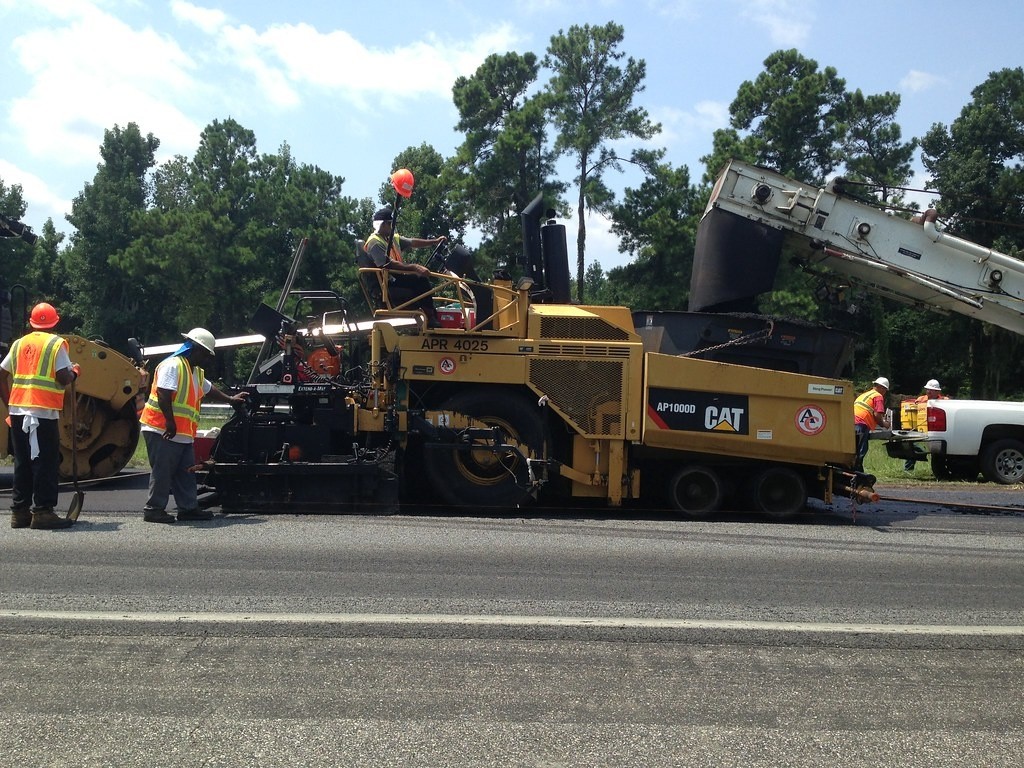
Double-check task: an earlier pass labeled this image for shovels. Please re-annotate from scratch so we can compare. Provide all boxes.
[65,380,85,521]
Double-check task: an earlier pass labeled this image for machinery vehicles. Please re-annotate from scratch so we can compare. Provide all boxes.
[0,214,150,482]
[188,158,1024,522]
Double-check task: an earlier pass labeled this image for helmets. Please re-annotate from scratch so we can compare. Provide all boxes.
[872,377,890,391]
[924,379,942,391]
[391,169,414,198]
[29,302,60,329]
[181,327,215,356]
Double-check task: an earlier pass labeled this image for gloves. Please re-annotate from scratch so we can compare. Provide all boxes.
[72,363,81,377]
[5,416,12,427]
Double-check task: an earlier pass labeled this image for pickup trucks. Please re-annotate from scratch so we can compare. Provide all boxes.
[868,399,1024,484]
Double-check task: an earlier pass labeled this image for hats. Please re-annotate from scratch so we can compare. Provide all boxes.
[373,209,393,235]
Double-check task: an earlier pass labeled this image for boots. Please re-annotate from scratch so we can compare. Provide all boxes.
[427,308,442,327]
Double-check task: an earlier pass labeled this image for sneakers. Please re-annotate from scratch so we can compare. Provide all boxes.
[177,508,214,520]
[144,510,175,523]
[11,509,72,529]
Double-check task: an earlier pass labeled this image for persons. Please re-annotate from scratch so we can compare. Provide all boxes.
[364,208,447,328]
[0,302,80,529]
[904,379,949,470]
[854,376,890,473]
[139,327,249,523]
